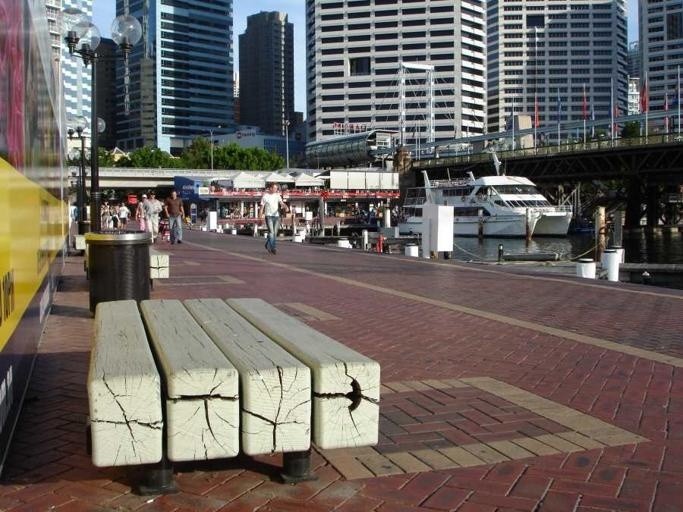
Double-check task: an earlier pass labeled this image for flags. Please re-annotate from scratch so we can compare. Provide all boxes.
[663,99,668,127]
[638,82,647,112]
[557,95,562,125]
[612,107,618,132]
[607,102,610,119]
[670,89,677,108]
[582,94,588,119]
[535,91,539,128]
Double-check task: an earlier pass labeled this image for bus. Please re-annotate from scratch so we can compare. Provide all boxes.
[300,128,400,168]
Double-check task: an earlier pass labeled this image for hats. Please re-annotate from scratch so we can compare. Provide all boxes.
[142,194,147,198]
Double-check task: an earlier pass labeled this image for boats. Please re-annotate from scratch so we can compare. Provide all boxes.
[567,192,683,231]
[502,251,559,260]
[395,172,575,239]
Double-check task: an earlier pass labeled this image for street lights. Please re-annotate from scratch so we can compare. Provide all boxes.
[52,4,142,282]
[202,125,222,171]
[62,113,107,257]
[69,165,90,221]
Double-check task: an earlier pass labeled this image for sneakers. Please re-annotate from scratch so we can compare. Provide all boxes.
[150,238,157,244]
[171,238,183,244]
[265,244,277,255]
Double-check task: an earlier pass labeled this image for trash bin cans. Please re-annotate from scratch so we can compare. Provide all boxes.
[84,229,152,314]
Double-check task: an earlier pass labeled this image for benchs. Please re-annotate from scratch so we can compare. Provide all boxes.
[86,297,380,495]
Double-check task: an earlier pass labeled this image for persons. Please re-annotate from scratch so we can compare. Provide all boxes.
[101,200,132,233]
[136,192,192,245]
[199,208,208,223]
[256,182,290,255]
[292,204,414,226]
[221,206,242,219]
